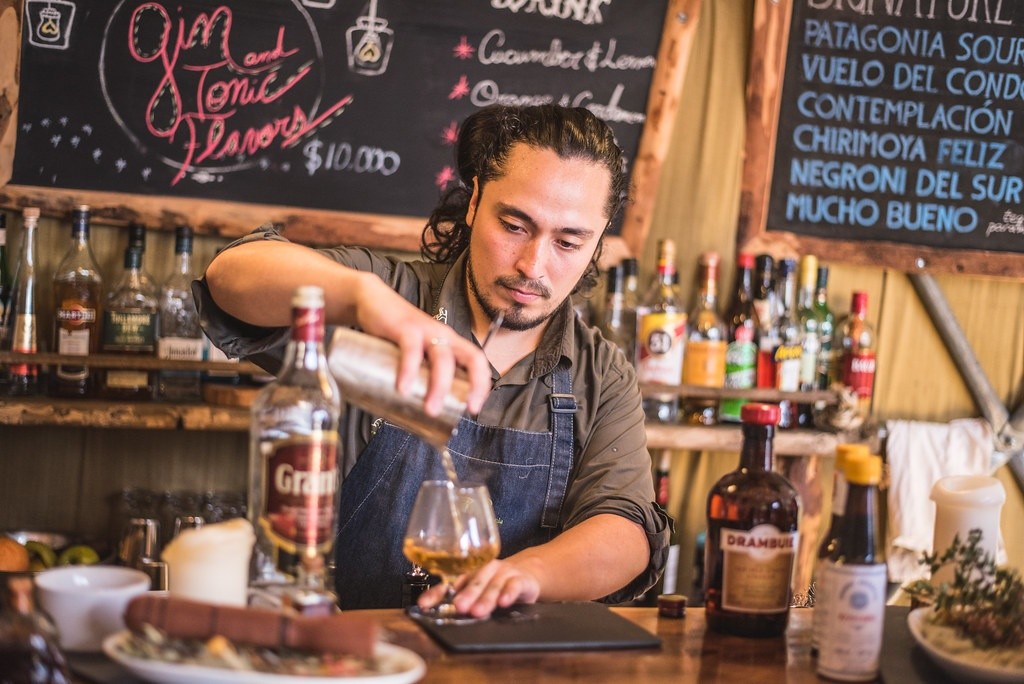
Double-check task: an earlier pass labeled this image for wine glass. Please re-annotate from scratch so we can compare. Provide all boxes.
[403,478,501,625]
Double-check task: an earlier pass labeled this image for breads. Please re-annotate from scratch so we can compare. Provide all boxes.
[121,594,381,660]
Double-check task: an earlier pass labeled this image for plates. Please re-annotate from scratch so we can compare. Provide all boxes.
[906,603,1024,684]
[101,631,428,684]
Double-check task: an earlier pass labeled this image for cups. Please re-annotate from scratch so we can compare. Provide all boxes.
[326,327,471,450]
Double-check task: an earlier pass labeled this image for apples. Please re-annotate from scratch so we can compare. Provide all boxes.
[24,540,100,572]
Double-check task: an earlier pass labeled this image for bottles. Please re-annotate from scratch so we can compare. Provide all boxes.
[808,441,872,659]
[638,450,681,608]
[592,237,877,436]
[246,286,345,602]
[814,453,887,683]
[0,202,276,408]
[704,402,803,638]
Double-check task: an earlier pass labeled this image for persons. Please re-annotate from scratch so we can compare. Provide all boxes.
[189,101,671,619]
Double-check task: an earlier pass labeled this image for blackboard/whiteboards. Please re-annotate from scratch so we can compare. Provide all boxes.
[734,0,1024,285]
[0,0,703,269]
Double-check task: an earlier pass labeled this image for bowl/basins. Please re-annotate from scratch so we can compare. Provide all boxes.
[35,565,152,652]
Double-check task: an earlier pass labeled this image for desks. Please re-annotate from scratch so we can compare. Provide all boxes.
[364,603,954,684]
